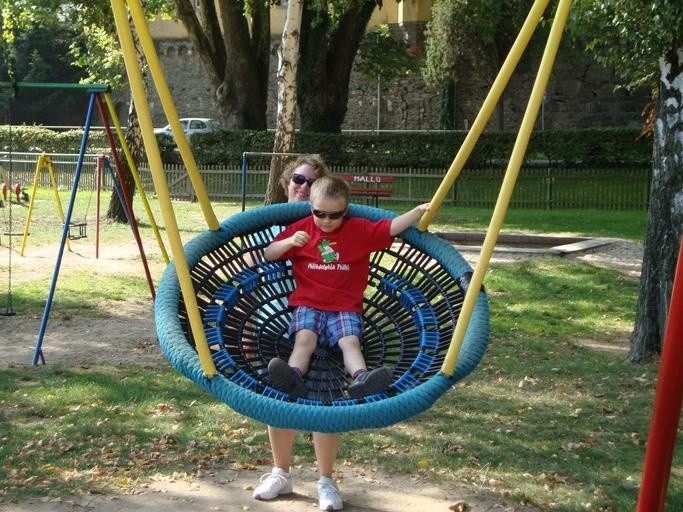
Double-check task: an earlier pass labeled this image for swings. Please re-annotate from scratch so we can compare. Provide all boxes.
[0,87,16,317]
[109,0,568,432]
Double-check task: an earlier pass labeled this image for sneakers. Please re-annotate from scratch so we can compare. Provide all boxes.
[253,466,293,500]
[347,365,394,399]
[267,356,308,398]
[316,476,344,512]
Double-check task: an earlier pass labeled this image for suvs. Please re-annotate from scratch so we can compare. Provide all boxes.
[152,117,215,144]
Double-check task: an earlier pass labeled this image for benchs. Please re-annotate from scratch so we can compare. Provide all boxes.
[335,174,393,207]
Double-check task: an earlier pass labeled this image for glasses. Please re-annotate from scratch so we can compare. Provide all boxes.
[291,173,315,187]
[312,209,346,220]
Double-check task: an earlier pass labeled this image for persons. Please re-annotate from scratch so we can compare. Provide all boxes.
[250,154,344,511]
[264,176,440,397]
